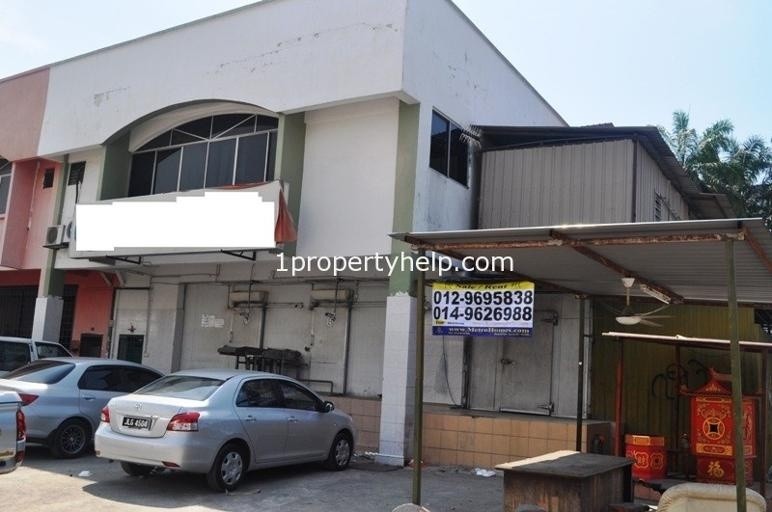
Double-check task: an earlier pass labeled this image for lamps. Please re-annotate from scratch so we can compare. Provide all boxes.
[616,316,642,325]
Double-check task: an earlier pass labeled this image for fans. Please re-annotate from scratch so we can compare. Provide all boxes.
[601,278,677,328]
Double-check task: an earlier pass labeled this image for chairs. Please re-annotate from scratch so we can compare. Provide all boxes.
[657,482,768,512]
[246,389,298,408]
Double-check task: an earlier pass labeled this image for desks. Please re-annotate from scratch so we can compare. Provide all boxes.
[494,450,637,512]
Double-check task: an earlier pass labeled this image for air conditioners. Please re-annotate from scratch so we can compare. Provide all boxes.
[42,224,69,249]
[62,221,72,244]
